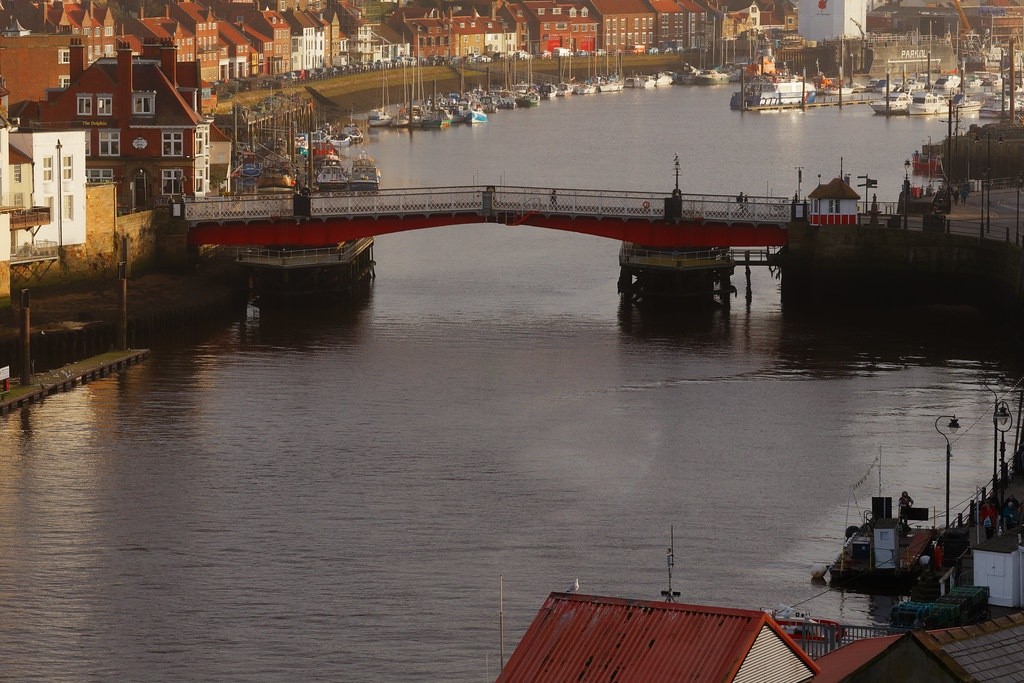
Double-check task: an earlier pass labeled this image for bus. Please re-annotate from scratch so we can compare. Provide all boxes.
[626,45,645,55]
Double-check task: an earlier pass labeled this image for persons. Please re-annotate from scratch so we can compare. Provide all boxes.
[980,492,1024,540]
[898,490,914,524]
[736,192,749,217]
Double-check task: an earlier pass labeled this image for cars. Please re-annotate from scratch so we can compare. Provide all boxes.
[645,46,685,55]
[212,48,606,84]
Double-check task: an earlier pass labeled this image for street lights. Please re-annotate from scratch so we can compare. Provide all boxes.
[795,167,804,203]
[56,139,64,248]
[935,414,961,529]
[974,133,1005,234]
[674,161,681,188]
[904,159,912,228]
[992,400,1013,532]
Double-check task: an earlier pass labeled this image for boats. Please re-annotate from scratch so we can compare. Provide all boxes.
[618,241,737,295]
[730,35,1024,116]
[829,497,936,582]
[760,602,848,643]
[421,85,516,127]
[237,122,381,190]
[624,69,677,89]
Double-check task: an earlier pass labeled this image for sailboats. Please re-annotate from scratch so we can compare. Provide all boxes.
[502,29,623,107]
[368,33,427,128]
[675,16,751,85]
